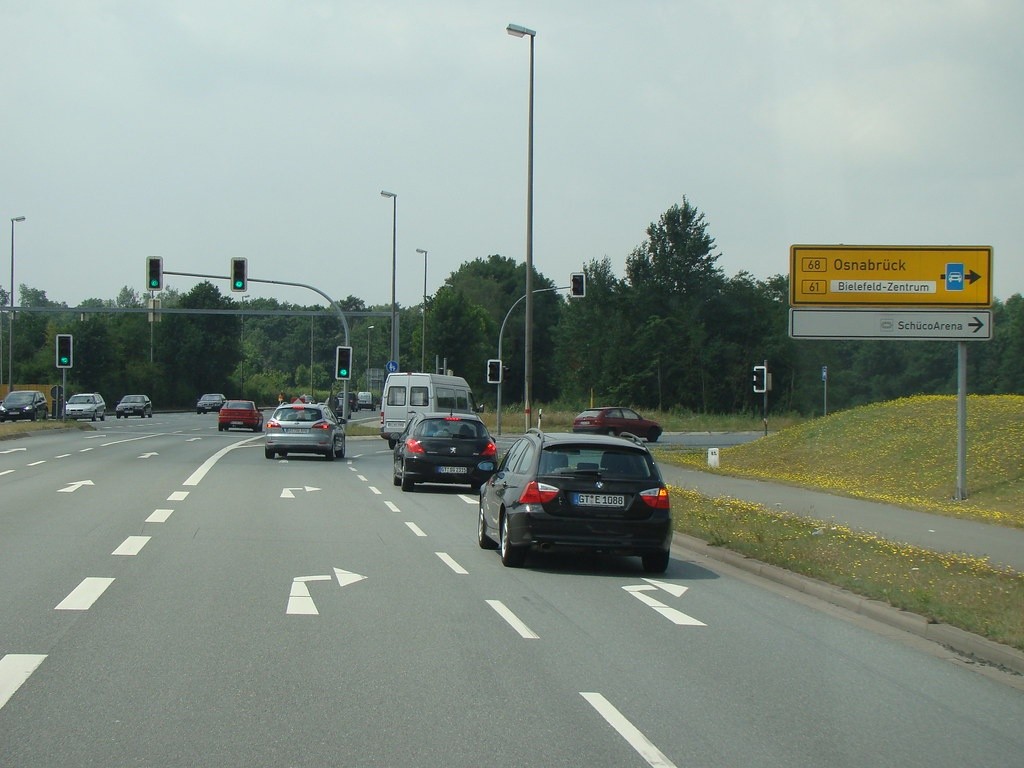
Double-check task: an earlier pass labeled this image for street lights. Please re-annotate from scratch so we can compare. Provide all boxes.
[366,190,428,391]
[8,216,26,392]
[240,295,250,400]
[506,24,536,434]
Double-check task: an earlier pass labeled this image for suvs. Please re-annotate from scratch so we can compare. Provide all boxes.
[61,392,106,422]
[478,428,673,574]
[573,406,663,442]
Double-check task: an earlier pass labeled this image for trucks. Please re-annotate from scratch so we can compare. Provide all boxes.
[380,372,481,449]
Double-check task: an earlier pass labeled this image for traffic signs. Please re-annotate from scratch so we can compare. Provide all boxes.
[789,245,994,340]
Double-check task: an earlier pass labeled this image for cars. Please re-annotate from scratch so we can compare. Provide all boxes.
[115,395,153,419]
[0,391,49,422]
[197,392,377,461]
[392,412,499,494]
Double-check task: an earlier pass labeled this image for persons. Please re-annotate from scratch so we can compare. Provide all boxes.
[278,390,285,402]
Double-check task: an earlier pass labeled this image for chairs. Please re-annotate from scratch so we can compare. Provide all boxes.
[311,414,320,420]
[601,452,630,478]
[287,413,297,419]
[542,453,568,473]
[458,424,473,437]
[424,423,438,435]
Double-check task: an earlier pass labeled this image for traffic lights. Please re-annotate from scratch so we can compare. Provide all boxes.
[570,272,586,298]
[335,346,353,380]
[751,366,767,393]
[146,256,163,291]
[55,334,73,368]
[487,360,503,383]
[231,257,248,292]
